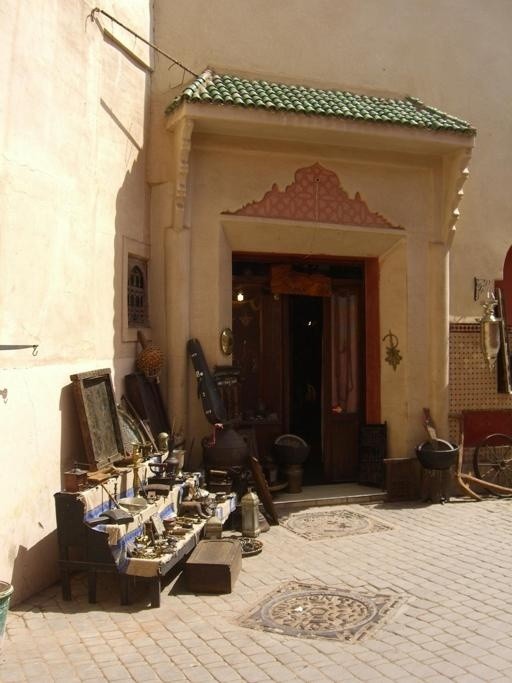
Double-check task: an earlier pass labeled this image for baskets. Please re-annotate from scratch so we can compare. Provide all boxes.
[138,344,164,379]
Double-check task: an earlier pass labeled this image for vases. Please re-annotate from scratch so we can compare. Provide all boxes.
[282,465,304,493]
[202,424,247,492]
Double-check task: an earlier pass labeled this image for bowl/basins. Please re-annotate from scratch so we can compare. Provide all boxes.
[270,444,311,465]
[415,442,459,469]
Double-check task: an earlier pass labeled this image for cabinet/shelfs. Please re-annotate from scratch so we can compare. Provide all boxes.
[54,450,237,608]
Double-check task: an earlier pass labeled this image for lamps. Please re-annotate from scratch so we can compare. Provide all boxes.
[237,289,244,303]
[474,276,501,374]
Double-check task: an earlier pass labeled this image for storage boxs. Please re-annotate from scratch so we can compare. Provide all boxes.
[184,539,242,593]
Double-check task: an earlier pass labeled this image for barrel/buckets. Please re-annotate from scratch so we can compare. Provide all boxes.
[0,580,14,647]
[420,468,449,505]
